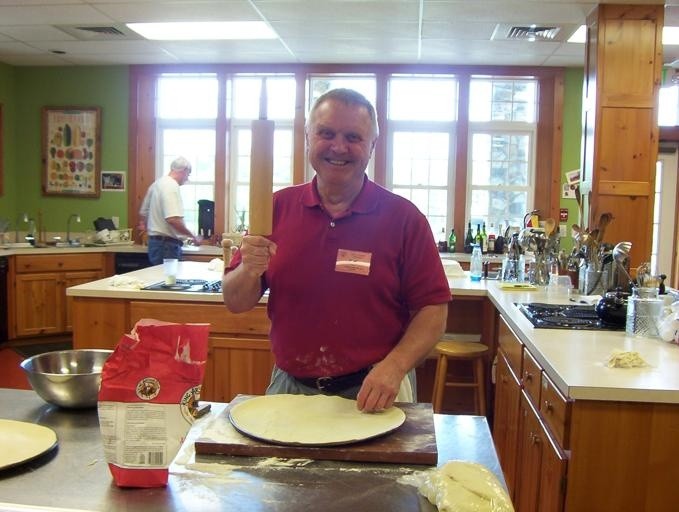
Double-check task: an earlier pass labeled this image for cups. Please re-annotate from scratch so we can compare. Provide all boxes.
[584,271,609,296]
[502,255,525,284]
[164,258,178,285]
[528,262,551,287]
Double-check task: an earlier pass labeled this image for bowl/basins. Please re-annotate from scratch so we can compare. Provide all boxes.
[19,349,115,408]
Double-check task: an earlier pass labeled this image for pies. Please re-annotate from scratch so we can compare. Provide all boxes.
[229,393,407,445]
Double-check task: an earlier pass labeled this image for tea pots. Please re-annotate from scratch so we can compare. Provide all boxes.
[597,285,631,326]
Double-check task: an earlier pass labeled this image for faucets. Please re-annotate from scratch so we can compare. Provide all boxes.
[15,212,28,242]
[67,214,80,241]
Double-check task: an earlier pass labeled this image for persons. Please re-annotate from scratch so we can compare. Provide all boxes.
[138,156,202,267]
[220,88,454,413]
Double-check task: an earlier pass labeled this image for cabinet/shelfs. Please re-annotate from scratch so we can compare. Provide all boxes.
[9,253,275,402]
[513,347,679,512]
[493,315,524,502]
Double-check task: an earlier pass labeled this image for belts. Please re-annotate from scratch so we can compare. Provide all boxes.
[148,236,183,247]
[292,365,373,395]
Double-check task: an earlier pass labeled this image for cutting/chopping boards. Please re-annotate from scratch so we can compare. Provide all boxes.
[195,393,438,465]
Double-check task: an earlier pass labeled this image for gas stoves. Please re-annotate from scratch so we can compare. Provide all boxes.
[512,302,626,331]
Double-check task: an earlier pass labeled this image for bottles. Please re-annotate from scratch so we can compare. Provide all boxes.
[626,286,664,337]
[508,232,520,260]
[470,243,483,281]
[438,222,504,254]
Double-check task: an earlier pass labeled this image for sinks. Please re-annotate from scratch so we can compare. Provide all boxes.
[51,242,106,248]
[2,246,50,249]
[482,271,500,279]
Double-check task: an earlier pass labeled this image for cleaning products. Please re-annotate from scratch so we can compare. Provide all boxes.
[469,242,482,282]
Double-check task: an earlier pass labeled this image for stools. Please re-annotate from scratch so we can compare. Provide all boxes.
[431,342,488,416]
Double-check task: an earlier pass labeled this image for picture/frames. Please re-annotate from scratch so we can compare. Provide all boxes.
[41,106,100,198]
[100,171,126,192]
